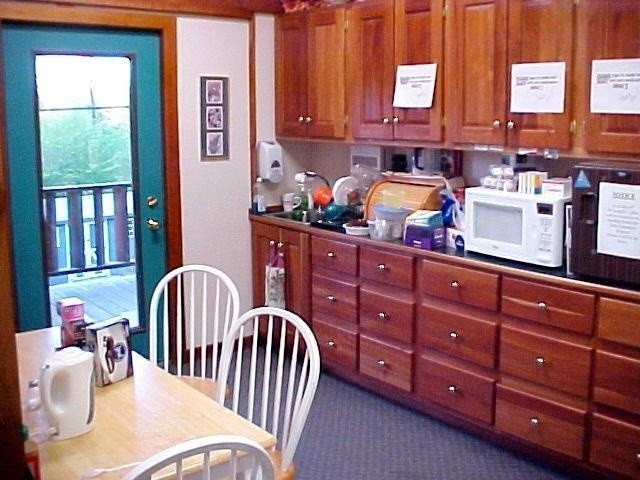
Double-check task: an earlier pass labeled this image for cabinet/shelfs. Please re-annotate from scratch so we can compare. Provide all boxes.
[578,1,639,172]
[357,241,415,409]
[588,284,639,480]
[310,230,361,392]
[501,273,597,472]
[445,1,578,158]
[421,252,499,450]
[272,2,347,144]
[349,1,445,149]
[249,215,310,369]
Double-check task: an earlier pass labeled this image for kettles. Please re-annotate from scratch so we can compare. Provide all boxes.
[38,345,97,442]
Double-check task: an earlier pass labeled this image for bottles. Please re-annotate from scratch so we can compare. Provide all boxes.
[307,189,314,210]
[24,379,48,445]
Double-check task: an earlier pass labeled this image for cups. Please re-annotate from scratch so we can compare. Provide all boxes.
[379,188,407,210]
[282,192,302,213]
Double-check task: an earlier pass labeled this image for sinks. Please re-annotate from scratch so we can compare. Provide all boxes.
[262,211,312,227]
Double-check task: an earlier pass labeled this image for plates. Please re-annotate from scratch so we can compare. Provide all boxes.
[342,223,370,236]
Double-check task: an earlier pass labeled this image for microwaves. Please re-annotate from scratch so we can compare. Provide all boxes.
[464,184,573,271]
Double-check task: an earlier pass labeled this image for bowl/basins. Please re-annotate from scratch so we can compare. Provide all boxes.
[331,177,360,205]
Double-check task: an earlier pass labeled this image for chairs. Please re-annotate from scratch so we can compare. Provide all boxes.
[115,432,280,480]
[214,302,324,480]
[147,262,243,404]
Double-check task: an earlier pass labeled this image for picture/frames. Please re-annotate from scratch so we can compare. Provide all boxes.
[197,72,233,164]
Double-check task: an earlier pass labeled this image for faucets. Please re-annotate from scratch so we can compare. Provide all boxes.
[306,170,332,196]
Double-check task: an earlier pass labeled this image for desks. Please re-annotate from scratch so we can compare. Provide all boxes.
[15,317,283,480]
